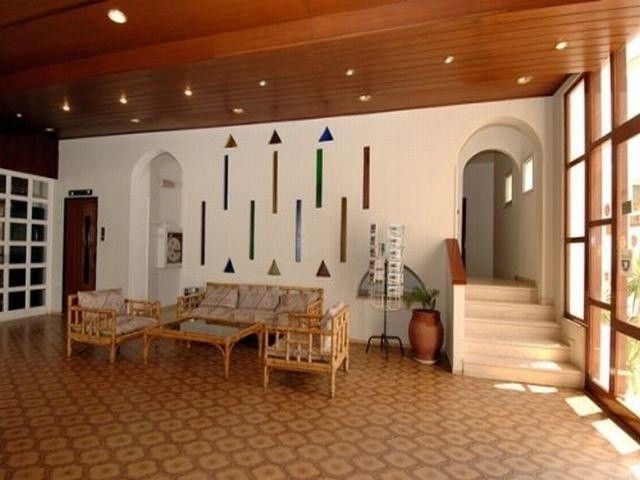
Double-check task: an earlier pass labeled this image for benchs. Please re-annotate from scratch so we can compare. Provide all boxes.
[176,282,323,344]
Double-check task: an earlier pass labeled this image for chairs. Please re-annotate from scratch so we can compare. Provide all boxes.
[262,306,349,397]
[67,294,160,364]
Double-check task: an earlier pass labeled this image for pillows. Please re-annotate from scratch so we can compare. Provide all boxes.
[315,302,346,352]
[199,285,319,314]
[78,288,127,323]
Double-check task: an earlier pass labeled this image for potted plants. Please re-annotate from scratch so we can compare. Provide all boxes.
[403,287,444,366]
